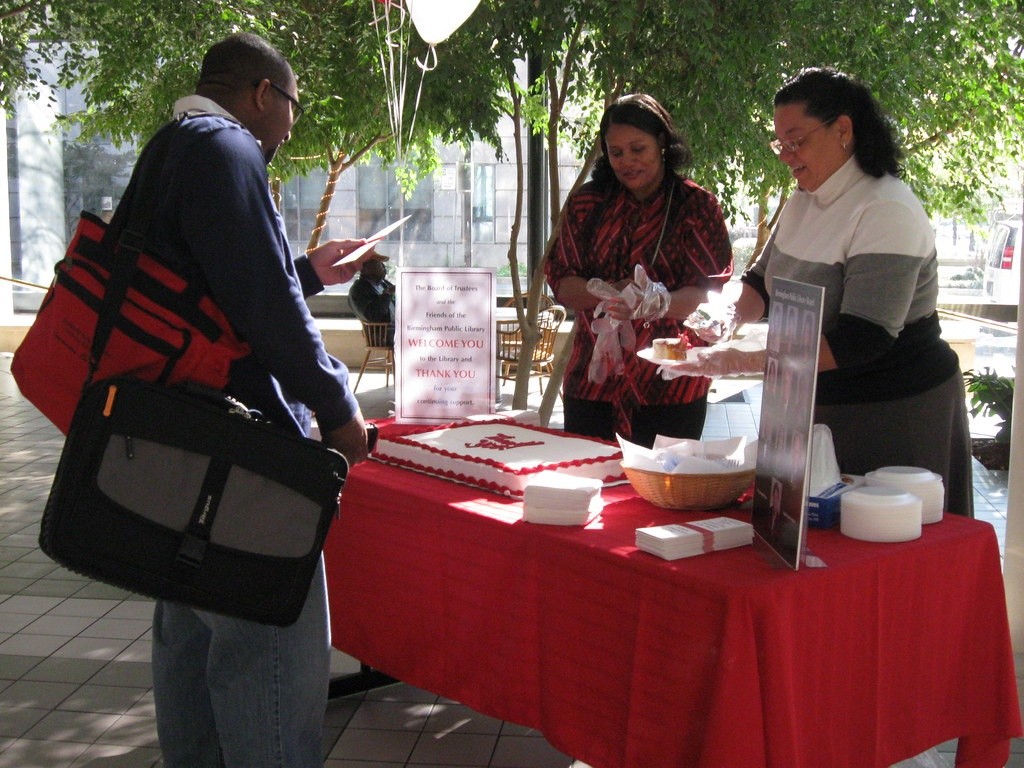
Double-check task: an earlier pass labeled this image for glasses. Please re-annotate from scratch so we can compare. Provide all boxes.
[254,79,304,125]
[769,113,840,153]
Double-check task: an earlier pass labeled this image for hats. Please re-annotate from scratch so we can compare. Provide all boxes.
[365,251,390,262]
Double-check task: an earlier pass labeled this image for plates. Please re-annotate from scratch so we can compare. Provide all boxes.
[836,465,947,544]
[637,344,703,367]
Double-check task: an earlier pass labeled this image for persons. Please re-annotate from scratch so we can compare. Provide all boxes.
[543,94,733,451]
[352,251,396,348]
[671,67,976,522]
[106,31,384,768]
[760,308,814,535]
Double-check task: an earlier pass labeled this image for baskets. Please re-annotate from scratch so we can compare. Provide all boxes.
[619,457,756,510]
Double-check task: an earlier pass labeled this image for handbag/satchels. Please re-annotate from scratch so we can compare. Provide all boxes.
[38,375,349,624]
[10,211,246,436]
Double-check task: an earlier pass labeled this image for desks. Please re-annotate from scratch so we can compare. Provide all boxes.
[497,307,527,403]
[325,417,1024,768]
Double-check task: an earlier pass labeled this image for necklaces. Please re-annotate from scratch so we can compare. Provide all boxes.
[643,177,675,328]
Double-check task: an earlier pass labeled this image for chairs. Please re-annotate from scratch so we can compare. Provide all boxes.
[353,316,394,393]
[497,304,566,402]
[496,293,555,395]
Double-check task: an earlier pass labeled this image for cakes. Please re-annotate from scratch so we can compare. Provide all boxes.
[370,418,627,495]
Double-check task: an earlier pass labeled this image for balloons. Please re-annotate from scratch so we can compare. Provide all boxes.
[406,0,481,46]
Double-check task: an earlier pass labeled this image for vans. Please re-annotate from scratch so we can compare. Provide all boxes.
[979,216,1022,307]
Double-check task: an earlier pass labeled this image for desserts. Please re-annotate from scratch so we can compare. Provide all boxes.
[652,328,688,361]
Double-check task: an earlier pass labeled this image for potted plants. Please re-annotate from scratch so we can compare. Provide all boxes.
[963,366,1015,471]
